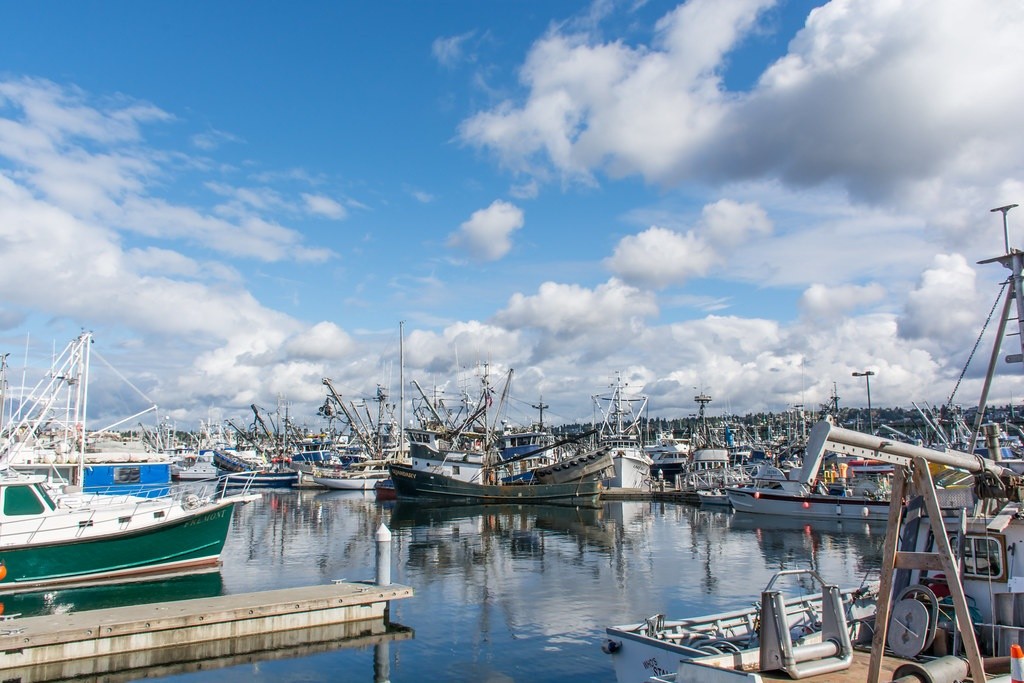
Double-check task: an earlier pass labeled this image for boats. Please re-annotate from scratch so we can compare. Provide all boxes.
[0,199,1024,683]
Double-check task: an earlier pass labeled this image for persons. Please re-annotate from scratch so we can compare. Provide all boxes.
[755,620,760,637]
